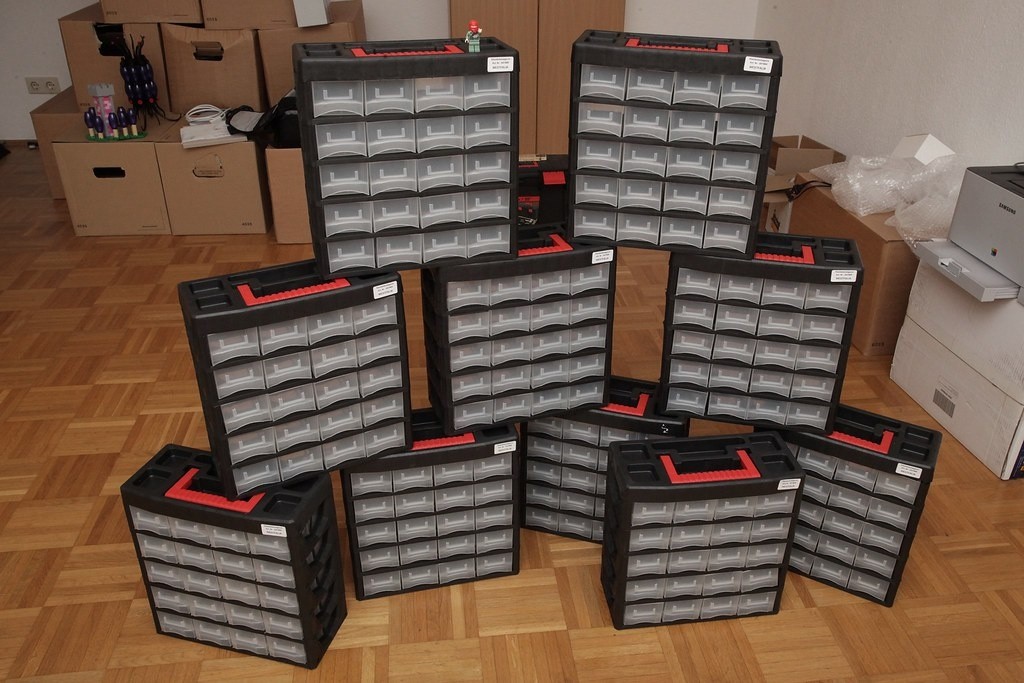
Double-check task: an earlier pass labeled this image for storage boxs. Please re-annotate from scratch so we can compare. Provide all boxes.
[755,133,1024,481]
[28,0,366,247]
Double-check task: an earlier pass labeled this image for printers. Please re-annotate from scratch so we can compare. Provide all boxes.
[914,164,1024,307]
